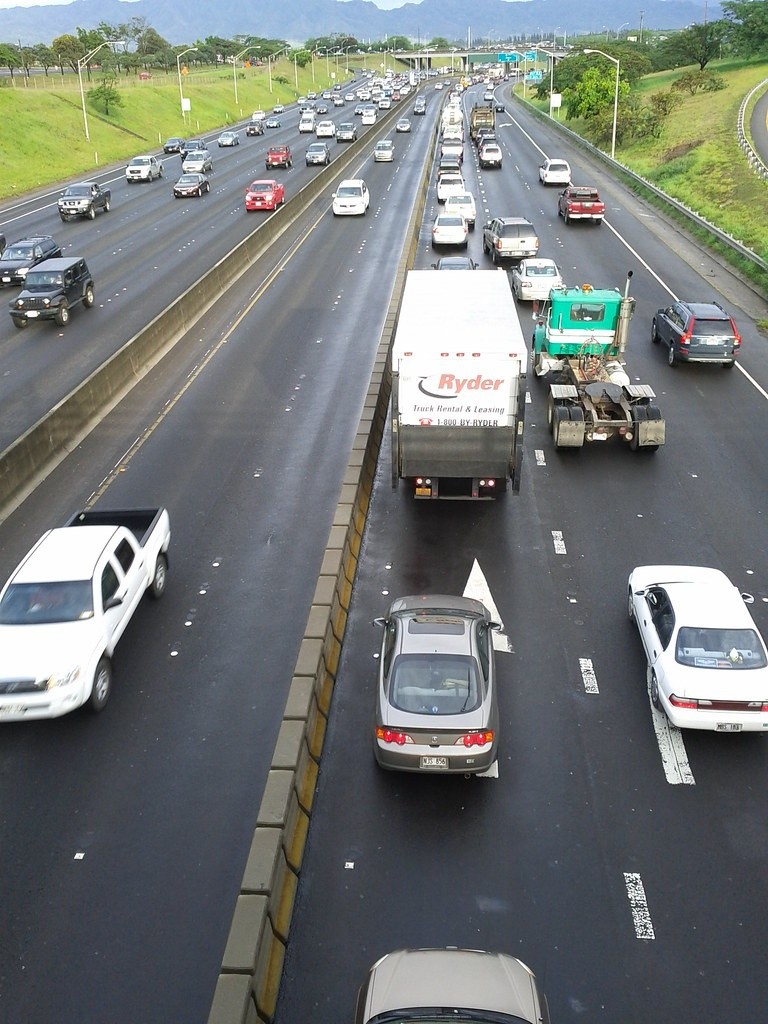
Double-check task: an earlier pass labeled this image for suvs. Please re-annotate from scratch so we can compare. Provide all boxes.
[651,299,743,368]
[9,256,96,328]
[0,230,64,289]
[482,216,539,266]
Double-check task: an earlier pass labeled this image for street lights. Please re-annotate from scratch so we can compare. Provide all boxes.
[311,46,327,84]
[336,46,350,77]
[346,44,357,74]
[531,48,554,118]
[295,50,311,89]
[325,46,339,78]
[602,26,608,42]
[538,27,543,43]
[451,49,462,77]
[617,23,630,40]
[510,51,526,100]
[268,47,291,94]
[584,48,620,161]
[176,47,199,124]
[554,27,561,52]
[234,45,261,104]
[78,40,126,142]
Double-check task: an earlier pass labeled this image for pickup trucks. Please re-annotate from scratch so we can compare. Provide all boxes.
[0,506,172,723]
[57,181,111,222]
[245,179,285,212]
[558,186,606,225]
[125,155,164,184]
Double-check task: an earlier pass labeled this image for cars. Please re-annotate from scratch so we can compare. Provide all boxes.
[395,118,412,133]
[359,45,479,55]
[539,158,572,187]
[373,593,502,779]
[626,564,768,737]
[431,256,480,270]
[510,258,563,303]
[139,70,153,80]
[354,945,550,1024]
[174,172,211,199]
[162,61,545,204]
[331,178,370,216]
[431,210,471,249]
[373,139,396,163]
[442,192,477,229]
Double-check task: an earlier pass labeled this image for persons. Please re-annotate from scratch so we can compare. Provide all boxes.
[53,275,62,284]
[31,587,74,610]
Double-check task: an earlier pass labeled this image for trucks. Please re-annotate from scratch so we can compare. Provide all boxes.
[390,267,529,501]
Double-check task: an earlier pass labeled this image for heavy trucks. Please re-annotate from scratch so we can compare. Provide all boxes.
[530,269,666,453]
